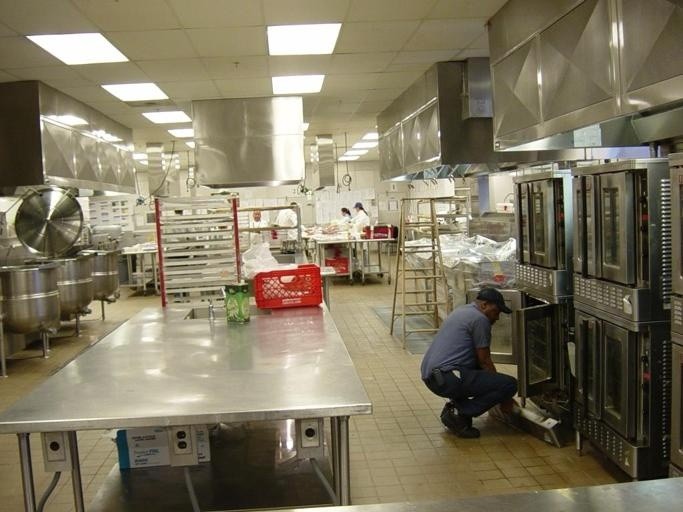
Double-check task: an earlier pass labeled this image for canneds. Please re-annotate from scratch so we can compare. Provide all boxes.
[225,283,248,325]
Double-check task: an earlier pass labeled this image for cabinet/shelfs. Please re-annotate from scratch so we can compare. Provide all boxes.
[154,197,241,307]
[414,240,515,324]
[416,196,469,238]
[469,212,514,242]
[205,205,301,267]
[573,160,667,484]
[666,160,682,479]
[511,172,571,425]
[75,195,129,230]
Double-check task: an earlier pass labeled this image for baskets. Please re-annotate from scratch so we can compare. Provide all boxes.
[254,264,322,308]
[326,256,349,273]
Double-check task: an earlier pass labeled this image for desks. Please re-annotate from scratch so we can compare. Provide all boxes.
[121,241,160,296]
[0,295,372,512]
[290,476,682,512]
[303,223,397,286]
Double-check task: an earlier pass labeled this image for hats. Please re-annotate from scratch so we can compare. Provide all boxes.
[353,202,362,208]
[478,288,513,314]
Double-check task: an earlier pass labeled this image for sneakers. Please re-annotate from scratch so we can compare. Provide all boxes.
[440,402,481,438]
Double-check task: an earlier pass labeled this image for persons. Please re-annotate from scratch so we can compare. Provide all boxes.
[338,203,370,265]
[164,210,197,303]
[248,202,314,241]
[421,289,518,438]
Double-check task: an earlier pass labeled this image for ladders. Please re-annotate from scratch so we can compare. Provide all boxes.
[390,197,450,350]
